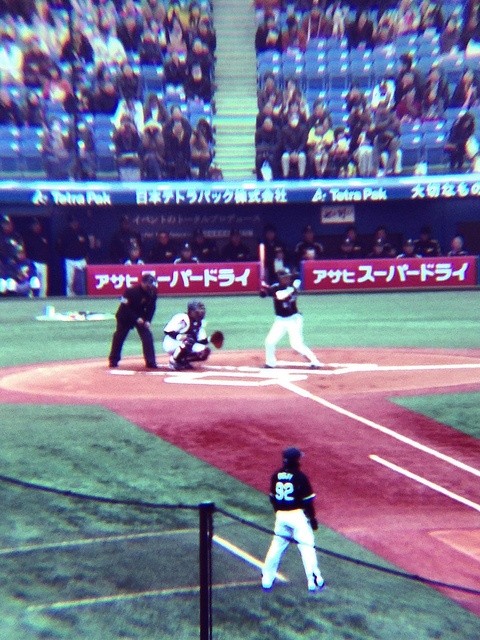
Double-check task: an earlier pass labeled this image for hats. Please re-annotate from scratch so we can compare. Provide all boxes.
[188,301,205,311]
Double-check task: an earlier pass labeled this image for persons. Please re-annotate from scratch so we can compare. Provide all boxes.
[297,224,326,260]
[183,62,211,103]
[282,15,306,52]
[397,86,421,122]
[264,226,296,279]
[258,446,327,594]
[338,226,364,259]
[152,231,174,263]
[65,79,93,115]
[191,18,215,54]
[329,127,352,177]
[446,112,475,168]
[161,301,217,369]
[373,79,396,109]
[306,101,332,130]
[348,7,374,40]
[175,240,199,264]
[116,217,140,237]
[258,73,282,109]
[0,34,24,84]
[282,77,306,105]
[422,67,450,105]
[167,0,188,27]
[144,94,169,122]
[27,216,50,299]
[256,118,281,181]
[113,116,142,180]
[94,62,116,109]
[190,119,213,179]
[375,102,400,132]
[352,110,373,177]
[439,15,460,52]
[446,234,472,257]
[163,53,183,95]
[255,12,282,52]
[113,93,143,128]
[423,82,444,120]
[188,39,211,63]
[123,238,145,266]
[108,275,158,369]
[345,83,367,112]
[394,54,423,102]
[425,1,443,31]
[283,88,307,118]
[94,26,127,62]
[0,212,25,260]
[451,69,480,108]
[264,267,320,369]
[43,117,71,180]
[190,229,223,262]
[374,8,395,43]
[23,92,46,129]
[118,64,142,99]
[60,28,94,64]
[347,103,372,128]
[1,245,40,297]
[25,37,50,85]
[372,132,403,177]
[141,0,167,21]
[54,0,119,26]
[1,90,22,127]
[326,2,351,37]
[307,118,335,176]
[166,119,191,180]
[140,20,165,63]
[121,0,141,51]
[365,228,395,259]
[43,65,73,101]
[166,17,187,53]
[185,2,210,33]
[256,102,280,129]
[61,216,94,296]
[282,112,308,177]
[142,119,165,180]
[396,238,423,258]
[302,0,327,38]
[224,228,252,261]
[417,224,442,257]
[282,100,307,123]
[396,1,421,35]
[72,119,97,181]
[268,89,277,107]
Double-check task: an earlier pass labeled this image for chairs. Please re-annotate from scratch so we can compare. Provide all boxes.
[0,47,214,179]
[255,0,480,176]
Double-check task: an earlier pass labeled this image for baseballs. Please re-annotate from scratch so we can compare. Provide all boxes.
[293,279,301,287]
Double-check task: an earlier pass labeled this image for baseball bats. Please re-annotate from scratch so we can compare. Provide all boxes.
[259,243,265,286]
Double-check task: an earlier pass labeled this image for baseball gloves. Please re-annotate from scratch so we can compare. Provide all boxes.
[209,331,223,349]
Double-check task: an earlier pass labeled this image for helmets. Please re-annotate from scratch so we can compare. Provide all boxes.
[140,274,157,298]
[282,448,301,466]
[276,268,293,278]
